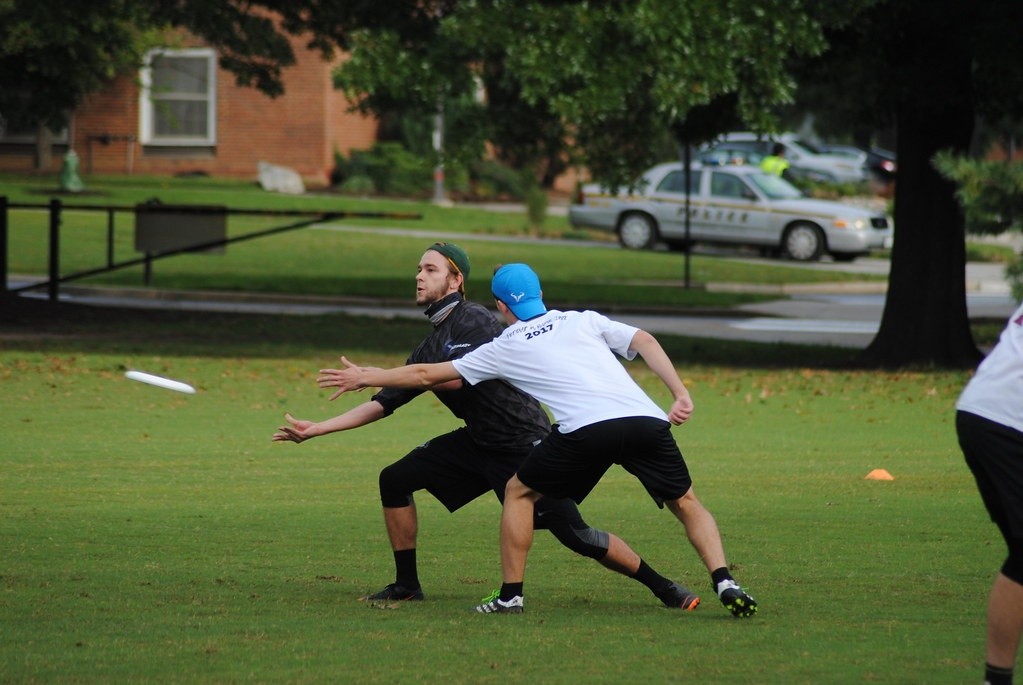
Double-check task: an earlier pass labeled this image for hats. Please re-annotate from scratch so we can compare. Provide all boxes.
[491,263,547,320]
[425,241,469,301]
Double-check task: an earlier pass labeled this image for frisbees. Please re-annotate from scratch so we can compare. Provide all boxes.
[124,371,196,395]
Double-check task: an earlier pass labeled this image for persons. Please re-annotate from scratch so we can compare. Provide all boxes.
[955,302,1023,685]
[272,241,700,609]
[724,141,808,192]
[317,262,760,620]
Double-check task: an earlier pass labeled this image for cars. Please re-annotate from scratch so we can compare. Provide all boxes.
[568,160,890,263]
[647,132,869,183]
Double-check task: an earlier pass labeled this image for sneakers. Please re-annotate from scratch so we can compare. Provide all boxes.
[357,582,424,604]
[711,578,758,619]
[470,588,524,616]
[666,583,701,611]
